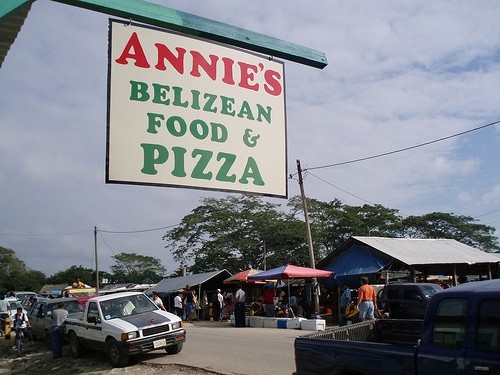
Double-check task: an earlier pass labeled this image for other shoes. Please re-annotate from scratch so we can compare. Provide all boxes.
[52,354,63,359]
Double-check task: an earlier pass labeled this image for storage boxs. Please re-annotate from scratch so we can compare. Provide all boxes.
[230,314,326,331]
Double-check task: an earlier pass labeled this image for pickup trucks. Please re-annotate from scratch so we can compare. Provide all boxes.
[61,291,188,368]
[291,278,500,375]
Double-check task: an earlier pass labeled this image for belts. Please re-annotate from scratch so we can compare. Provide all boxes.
[362,300,373,302]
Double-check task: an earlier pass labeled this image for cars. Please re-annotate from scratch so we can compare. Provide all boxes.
[6,290,84,343]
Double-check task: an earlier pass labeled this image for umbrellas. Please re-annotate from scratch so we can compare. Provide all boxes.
[223,263,334,307]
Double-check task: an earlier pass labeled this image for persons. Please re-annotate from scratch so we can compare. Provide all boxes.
[72,277,85,289]
[149,275,390,328]
[0,292,39,351]
[49,302,68,360]
